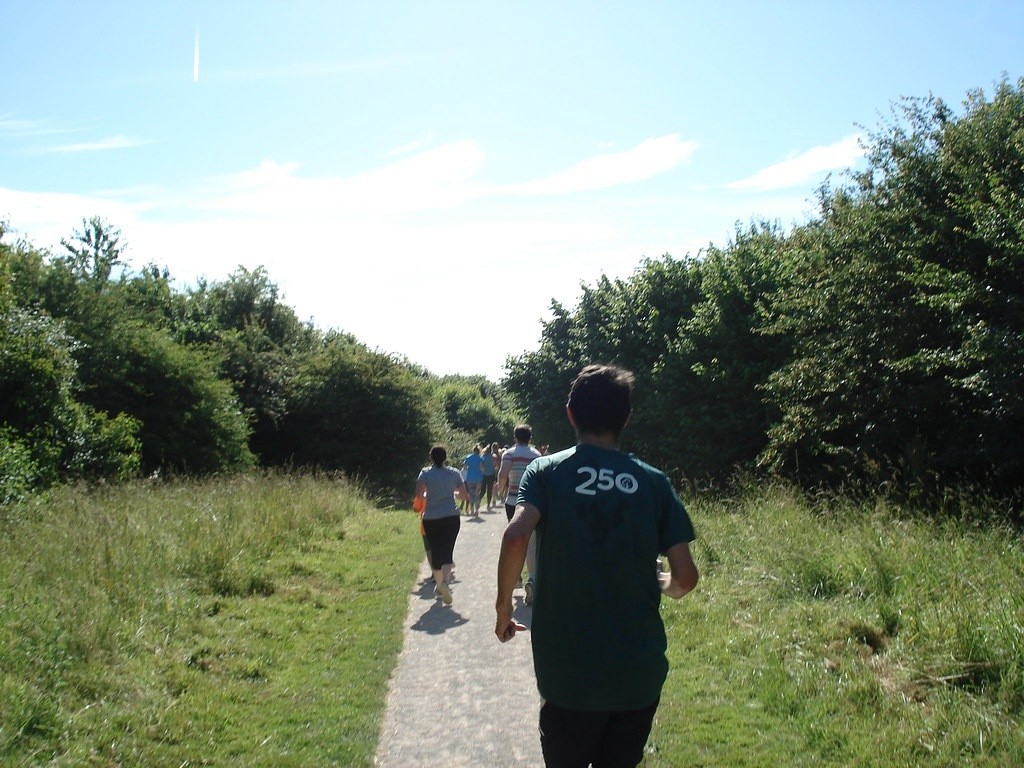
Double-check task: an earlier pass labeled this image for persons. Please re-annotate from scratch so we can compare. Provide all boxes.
[414,426,550,606]
[496,365,699,768]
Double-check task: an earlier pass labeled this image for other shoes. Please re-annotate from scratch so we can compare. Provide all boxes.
[437,582,453,605]
[524,578,535,606]
